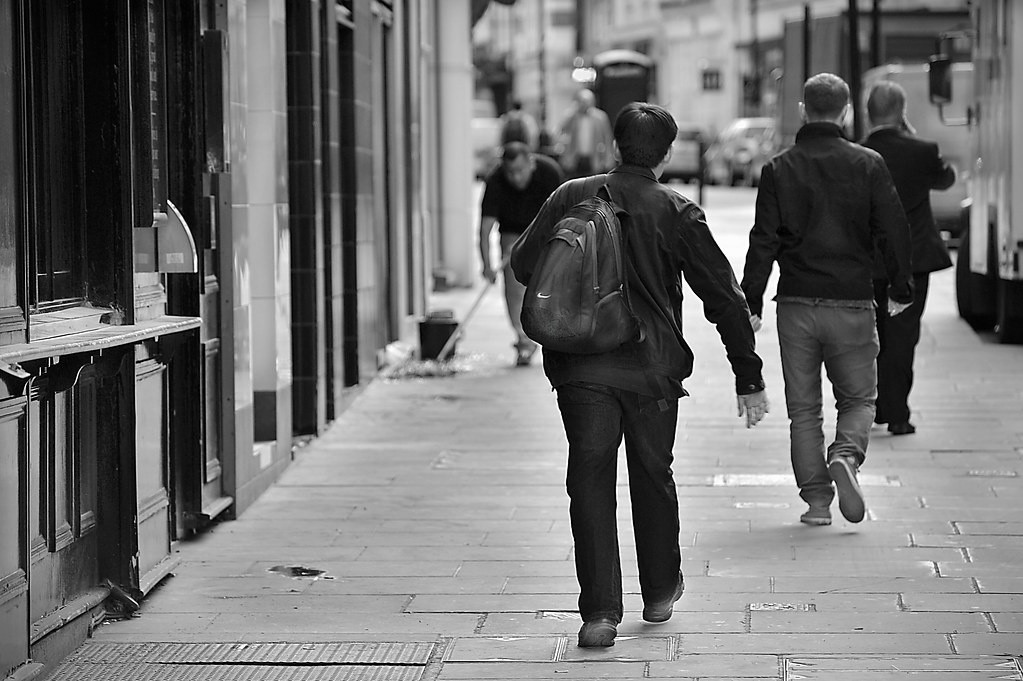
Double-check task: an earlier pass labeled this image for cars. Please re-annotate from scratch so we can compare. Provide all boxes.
[657,120,782,183]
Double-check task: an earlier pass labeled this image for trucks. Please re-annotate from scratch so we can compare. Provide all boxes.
[927,0,1023,347]
[851,60,979,243]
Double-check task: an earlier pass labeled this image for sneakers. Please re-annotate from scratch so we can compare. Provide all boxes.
[800,508,832,525]
[577,617,618,648]
[828,453,865,523]
[642,570,685,622]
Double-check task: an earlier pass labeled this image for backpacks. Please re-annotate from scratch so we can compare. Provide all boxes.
[518,173,648,353]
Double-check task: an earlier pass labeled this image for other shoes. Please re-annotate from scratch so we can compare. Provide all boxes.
[517,343,537,364]
[888,423,915,434]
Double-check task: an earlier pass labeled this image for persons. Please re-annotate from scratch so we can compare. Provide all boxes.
[556,89,615,179]
[854,80,961,436]
[510,102,771,650]
[501,101,561,161]
[740,72,918,526]
[478,143,566,369]
